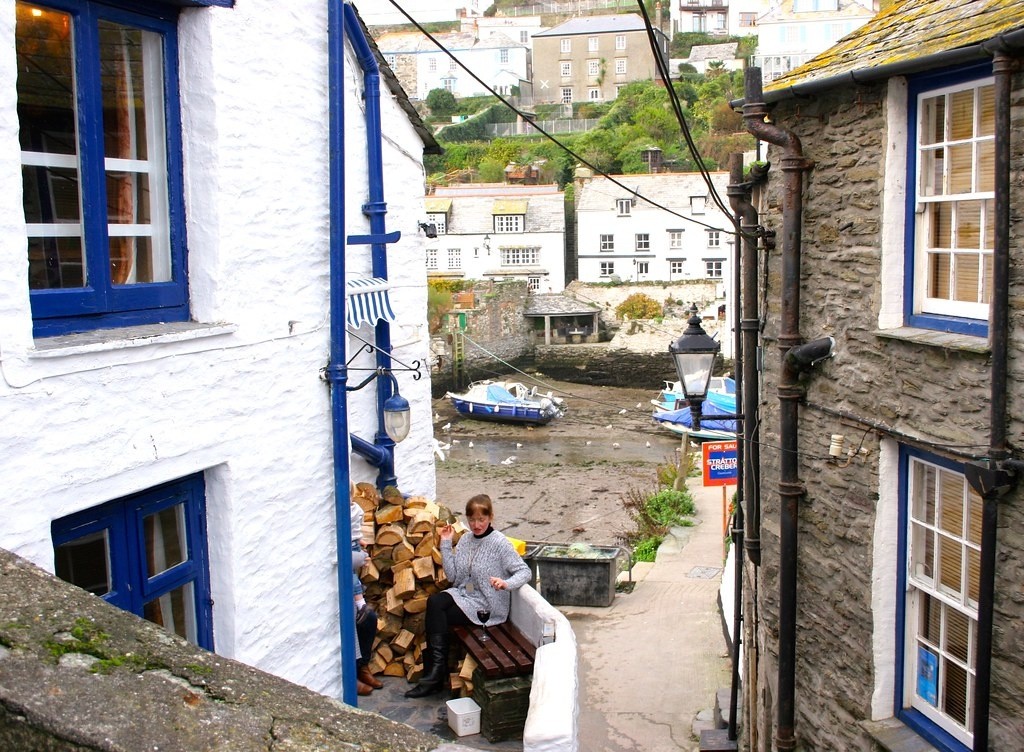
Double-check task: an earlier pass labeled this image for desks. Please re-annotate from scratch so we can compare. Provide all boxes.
[568,331,584,344]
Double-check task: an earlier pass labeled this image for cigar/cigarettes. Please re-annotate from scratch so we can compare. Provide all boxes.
[446,520,449,526]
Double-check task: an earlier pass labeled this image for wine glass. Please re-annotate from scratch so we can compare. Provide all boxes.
[477,605,490,642]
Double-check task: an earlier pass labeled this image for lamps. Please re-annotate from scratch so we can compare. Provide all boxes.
[483,233,491,255]
[347,369,412,445]
[430,340,447,366]
[669,302,754,431]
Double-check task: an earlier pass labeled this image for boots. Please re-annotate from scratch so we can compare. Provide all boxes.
[417,632,449,689]
[405,648,443,698]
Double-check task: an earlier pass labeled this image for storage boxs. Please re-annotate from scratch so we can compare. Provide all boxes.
[445,697,481,736]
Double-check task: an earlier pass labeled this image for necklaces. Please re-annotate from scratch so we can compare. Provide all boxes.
[466,532,483,592]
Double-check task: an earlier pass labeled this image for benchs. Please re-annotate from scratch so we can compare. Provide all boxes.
[453,619,540,746]
[553,328,566,343]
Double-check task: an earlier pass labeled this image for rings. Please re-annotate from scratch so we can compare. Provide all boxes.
[496,581,500,585]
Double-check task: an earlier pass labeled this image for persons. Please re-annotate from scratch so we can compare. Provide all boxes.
[349,479,384,695]
[404,493,533,699]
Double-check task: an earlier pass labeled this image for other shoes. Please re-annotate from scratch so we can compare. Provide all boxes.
[356,604,372,624]
[358,665,384,688]
[357,679,374,695]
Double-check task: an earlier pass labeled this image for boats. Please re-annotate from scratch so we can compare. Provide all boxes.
[651,375,736,440]
[446,379,566,426]
